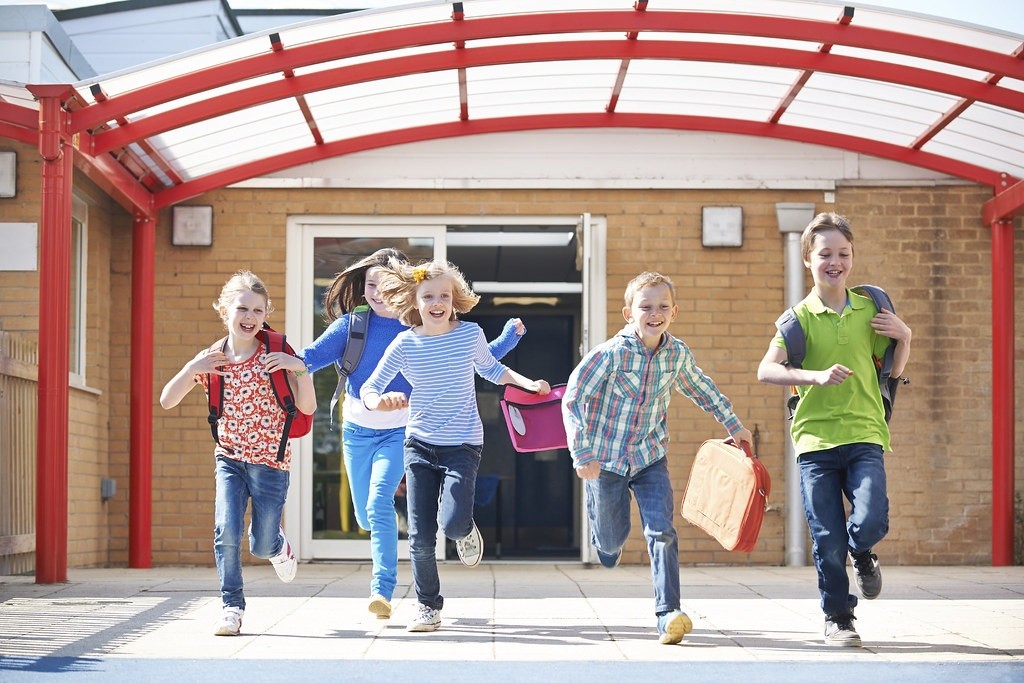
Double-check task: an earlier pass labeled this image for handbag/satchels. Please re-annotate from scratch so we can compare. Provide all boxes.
[500,383,568,453]
[680,437,771,553]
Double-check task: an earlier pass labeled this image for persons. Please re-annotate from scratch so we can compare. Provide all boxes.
[296,247,527,619]
[756,211,912,647]
[160,270,317,635]
[357,254,552,632]
[561,271,753,645]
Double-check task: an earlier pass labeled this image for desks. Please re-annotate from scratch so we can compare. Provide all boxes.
[447,474,516,560]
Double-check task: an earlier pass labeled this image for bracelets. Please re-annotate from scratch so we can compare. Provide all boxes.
[294,366,308,377]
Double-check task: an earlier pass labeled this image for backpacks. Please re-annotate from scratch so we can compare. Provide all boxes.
[207,323,315,461]
[775,284,900,425]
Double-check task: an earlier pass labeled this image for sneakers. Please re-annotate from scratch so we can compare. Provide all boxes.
[214,607,244,636]
[408,604,442,632]
[657,609,692,644]
[269,525,297,583]
[368,597,392,619]
[825,615,862,647]
[456,518,484,569]
[848,550,882,600]
[596,549,622,569]
